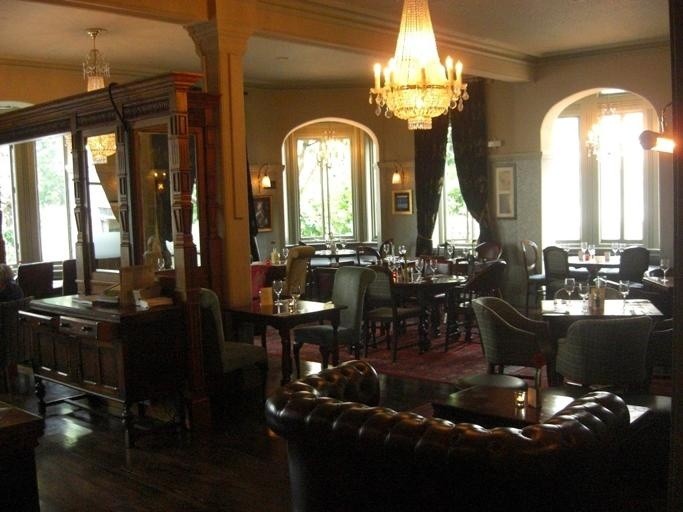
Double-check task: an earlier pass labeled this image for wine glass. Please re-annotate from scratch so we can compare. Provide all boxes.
[660,259,670,283]
[157,257,165,272]
[381,242,439,282]
[324,237,346,255]
[564,274,632,312]
[281,247,289,265]
[447,243,455,261]
[273,279,284,306]
[290,284,301,313]
[462,248,479,260]
[612,241,626,260]
[581,241,596,262]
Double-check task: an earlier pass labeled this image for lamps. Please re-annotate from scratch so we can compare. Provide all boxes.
[81,26,116,163]
[636,99,682,155]
[255,160,271,195]
[389,159,405,185]
[366,0,469,132]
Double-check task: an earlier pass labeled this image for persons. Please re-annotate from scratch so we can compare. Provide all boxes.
[0,263,24,299]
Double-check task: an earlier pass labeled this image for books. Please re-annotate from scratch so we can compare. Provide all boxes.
[71,282,121,305]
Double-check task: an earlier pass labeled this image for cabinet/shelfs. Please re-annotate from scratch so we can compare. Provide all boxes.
[18,291,188,449]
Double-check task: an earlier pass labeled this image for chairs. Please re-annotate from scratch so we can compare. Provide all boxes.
[17,260,53,300]
[58,259,100,296]
[199,238,683,512]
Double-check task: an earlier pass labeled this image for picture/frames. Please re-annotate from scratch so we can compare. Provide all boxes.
[251,195,273,233]
[490,161,517,220]
[390,189,412,214]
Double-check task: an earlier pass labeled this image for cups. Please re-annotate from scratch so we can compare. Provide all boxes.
[514,389,527,408]
[604,251,610,262]
[260,287,273,306]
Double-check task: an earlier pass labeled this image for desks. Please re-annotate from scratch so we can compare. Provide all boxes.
[0,399,44,512]
